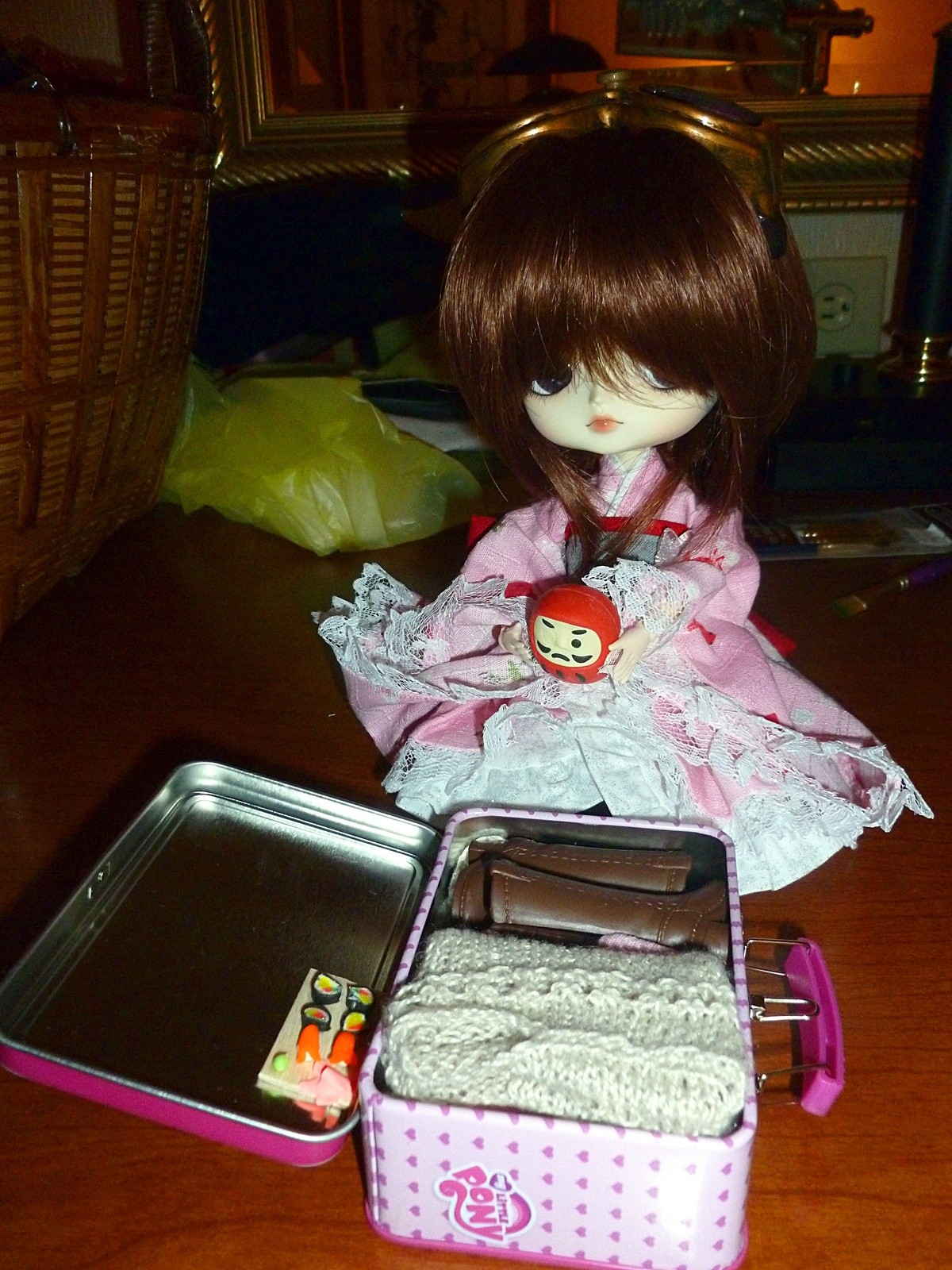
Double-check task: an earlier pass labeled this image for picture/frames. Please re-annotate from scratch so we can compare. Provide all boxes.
[228,0,550,153]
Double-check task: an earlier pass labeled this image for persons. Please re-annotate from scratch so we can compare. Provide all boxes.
[310,122,937,898]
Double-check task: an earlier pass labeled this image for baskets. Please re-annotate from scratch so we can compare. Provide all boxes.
[0,0,230,644]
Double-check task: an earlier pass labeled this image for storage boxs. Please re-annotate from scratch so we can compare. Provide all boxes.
[0,761,844,1270]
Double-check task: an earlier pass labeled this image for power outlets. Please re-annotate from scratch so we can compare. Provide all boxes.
[803,255,886,355]
